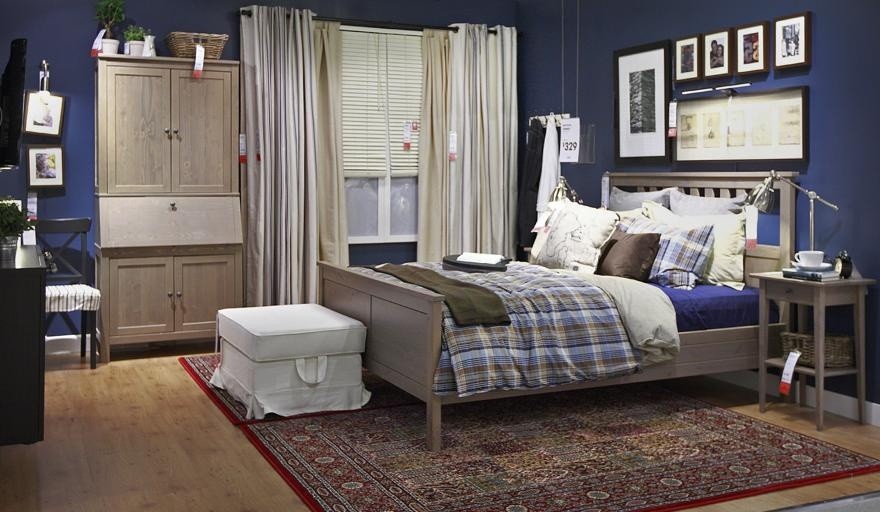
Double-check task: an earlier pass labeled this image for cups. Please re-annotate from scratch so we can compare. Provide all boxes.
[794,251,825,266]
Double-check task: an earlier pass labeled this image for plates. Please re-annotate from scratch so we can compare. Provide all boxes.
[793,263,833,272]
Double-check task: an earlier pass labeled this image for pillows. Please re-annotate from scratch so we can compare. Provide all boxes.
[526,186,753,293]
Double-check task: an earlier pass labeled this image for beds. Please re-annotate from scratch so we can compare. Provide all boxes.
[314,168,797,455]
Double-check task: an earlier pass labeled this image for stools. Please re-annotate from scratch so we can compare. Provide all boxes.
[217,302,367,424]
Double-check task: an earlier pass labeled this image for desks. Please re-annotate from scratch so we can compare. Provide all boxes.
[0,245,48,445]
[748,271,876,433]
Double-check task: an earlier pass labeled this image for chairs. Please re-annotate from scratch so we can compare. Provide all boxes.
[34,218,100,369]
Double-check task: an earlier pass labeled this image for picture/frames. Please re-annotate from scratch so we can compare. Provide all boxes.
[671,9,811,84]
[24,90,69,194]
[611,38,674,168]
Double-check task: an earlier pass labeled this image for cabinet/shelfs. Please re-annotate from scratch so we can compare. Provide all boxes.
[90,51,246,364]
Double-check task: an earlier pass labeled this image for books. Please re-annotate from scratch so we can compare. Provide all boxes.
[783,265,841,278]
[784,273,840,283]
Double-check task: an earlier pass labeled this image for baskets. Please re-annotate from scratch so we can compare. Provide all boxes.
[781,332,854,368]
[164,31,229,59]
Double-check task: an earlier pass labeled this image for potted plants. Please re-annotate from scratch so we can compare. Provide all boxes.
[1,201,39,249]
[94,0,154,55]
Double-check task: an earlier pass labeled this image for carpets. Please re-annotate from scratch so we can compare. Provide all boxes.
[177,354,878,512]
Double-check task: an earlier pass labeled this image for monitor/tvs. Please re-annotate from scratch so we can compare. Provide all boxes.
[0,37,28,172]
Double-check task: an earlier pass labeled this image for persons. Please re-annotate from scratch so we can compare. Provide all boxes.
[683,40,725,73]
[681,115,696,141]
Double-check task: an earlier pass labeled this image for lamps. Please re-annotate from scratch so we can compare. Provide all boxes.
[681,82,752,97]
[745,169,841,253]
[557,2,599,165]
